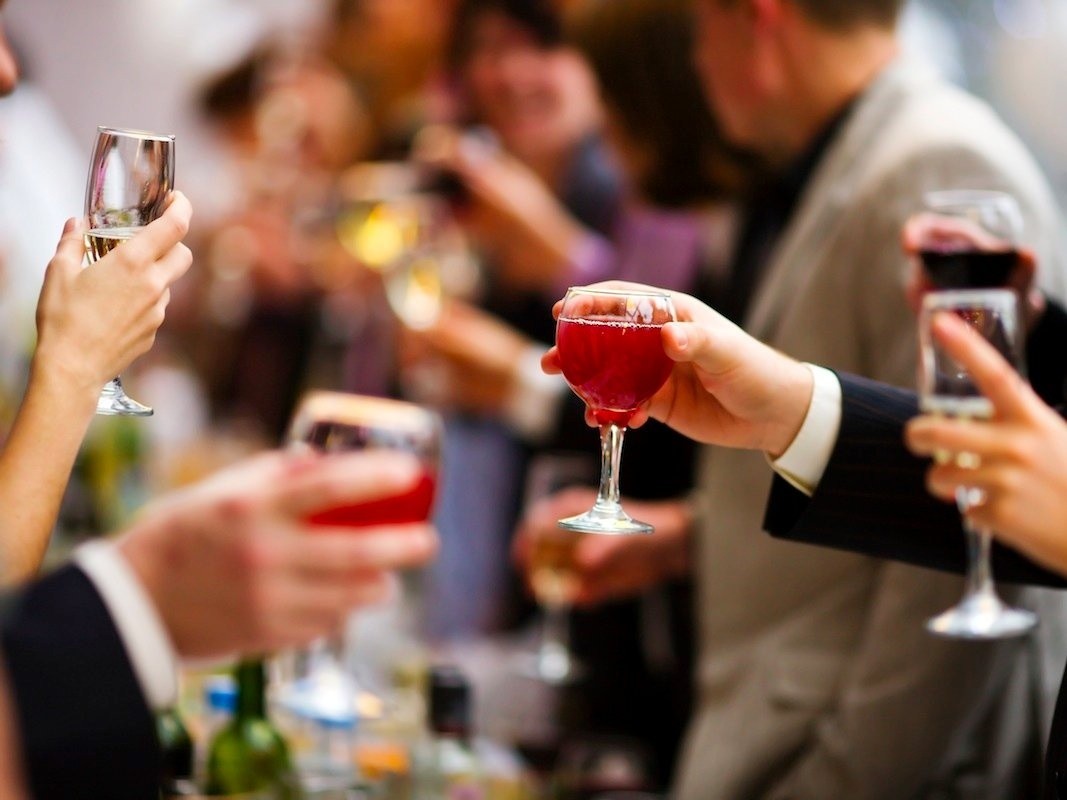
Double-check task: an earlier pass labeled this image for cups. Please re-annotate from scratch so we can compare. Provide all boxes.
[918,191,1025,291]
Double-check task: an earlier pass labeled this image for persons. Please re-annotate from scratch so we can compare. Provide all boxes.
[0,1,1066,800]
[0,192,191,602]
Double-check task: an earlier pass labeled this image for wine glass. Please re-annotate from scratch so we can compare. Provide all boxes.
[265,388,446,730]
[554,286,681,536]
[504,508,586,684]
[914,289,1039,642]
[82,127,176,416]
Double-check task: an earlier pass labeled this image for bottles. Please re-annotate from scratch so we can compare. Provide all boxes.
[200,648,308,800]
